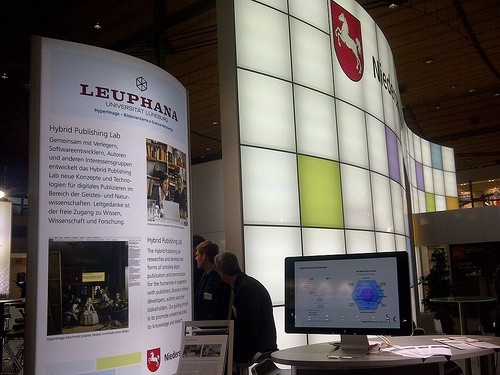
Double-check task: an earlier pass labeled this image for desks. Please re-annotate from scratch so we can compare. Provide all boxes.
[270,336,500,375]
[0,299,25,317]
[429,296,495,335]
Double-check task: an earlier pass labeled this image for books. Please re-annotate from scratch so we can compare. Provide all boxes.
[147,144,186,200]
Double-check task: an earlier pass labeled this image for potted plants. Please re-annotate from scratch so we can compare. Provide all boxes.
[411,249,453,334]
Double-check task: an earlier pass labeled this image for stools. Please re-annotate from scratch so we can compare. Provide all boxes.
[232,349,292,375]
[2,314,25,374]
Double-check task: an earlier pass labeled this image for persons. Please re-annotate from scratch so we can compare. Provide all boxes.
[192,235,206,292]
[174,176,188,218]
[213,253,278,375]
[70,286,123,328]
[195,241,230,329]
[150,174,172,208]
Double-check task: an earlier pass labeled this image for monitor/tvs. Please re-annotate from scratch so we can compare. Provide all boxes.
[284,251,412,358]
